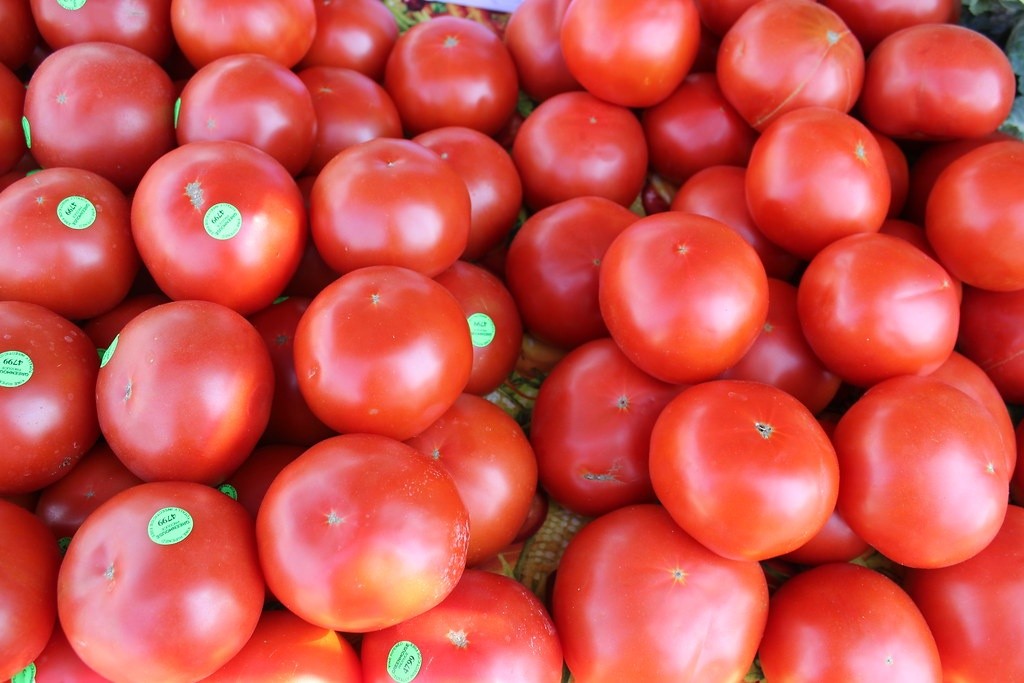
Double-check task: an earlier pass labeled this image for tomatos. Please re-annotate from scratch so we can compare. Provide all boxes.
[1,1,1024,683]
